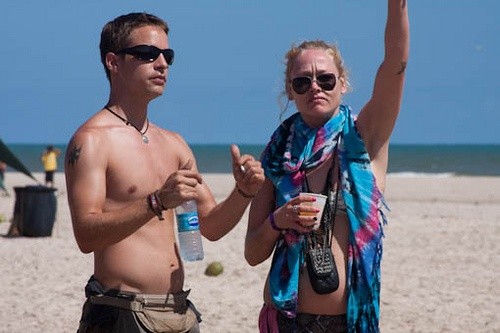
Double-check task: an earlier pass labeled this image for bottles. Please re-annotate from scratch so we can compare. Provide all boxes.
[175,199,204,261]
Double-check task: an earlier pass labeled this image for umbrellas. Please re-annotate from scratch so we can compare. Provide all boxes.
[0,139,38,182]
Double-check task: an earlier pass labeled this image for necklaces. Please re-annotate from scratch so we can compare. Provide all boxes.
[103,104,150,142]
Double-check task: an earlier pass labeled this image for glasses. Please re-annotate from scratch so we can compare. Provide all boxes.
[289,73,336,94]
[113,45,175,66]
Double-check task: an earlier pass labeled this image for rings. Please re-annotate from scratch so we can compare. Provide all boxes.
[239,165,246,173]
[292,205,298,213]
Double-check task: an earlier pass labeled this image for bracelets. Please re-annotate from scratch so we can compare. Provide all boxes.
[235,185,256,198]
[268,213,283,231]
[147,191,165,220]
[154,191,167,211]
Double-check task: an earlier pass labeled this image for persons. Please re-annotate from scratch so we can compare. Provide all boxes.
[64,12,266,332]
[0,161,10,197]
[245,0,411,333]
[41,146,61,188]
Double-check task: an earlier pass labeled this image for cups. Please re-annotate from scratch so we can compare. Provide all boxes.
[300,193,327,230]
